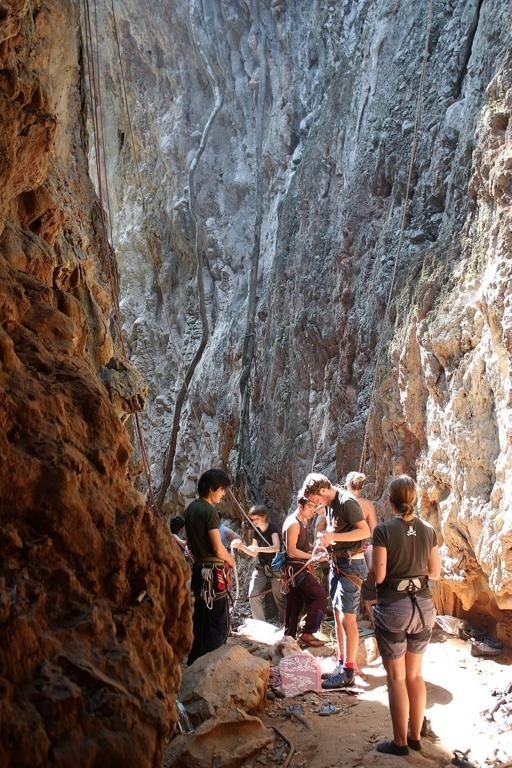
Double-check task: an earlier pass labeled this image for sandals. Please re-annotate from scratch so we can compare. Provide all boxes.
[300,636,324,647]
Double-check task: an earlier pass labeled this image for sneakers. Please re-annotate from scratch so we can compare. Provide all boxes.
[459,626,503,657]
[320,661,355,689]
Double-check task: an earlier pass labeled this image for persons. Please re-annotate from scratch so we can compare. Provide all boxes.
[364,475,441,755]
